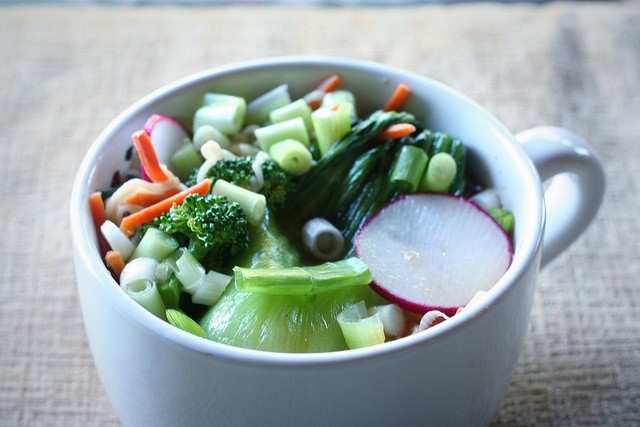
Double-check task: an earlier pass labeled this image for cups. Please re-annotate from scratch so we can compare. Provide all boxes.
[69,58,608,427]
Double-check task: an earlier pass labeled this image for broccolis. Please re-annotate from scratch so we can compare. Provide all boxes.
[185,154,297,210]
[138,192,248,310]
